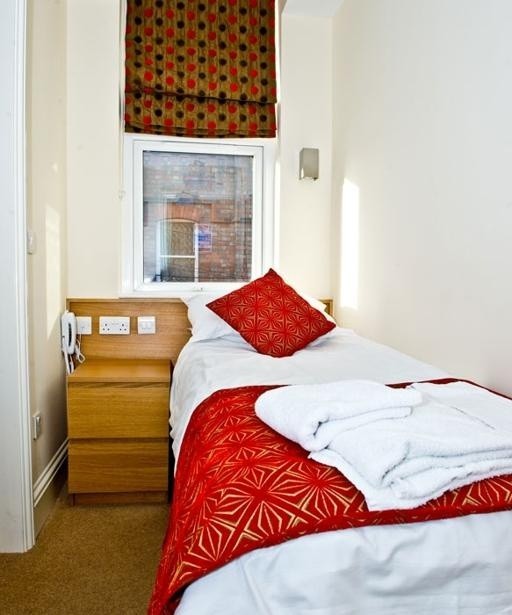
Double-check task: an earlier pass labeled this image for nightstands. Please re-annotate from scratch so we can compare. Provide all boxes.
[66,358,172,506]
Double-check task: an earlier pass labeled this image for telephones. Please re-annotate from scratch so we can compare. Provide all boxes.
[60,311,78,376]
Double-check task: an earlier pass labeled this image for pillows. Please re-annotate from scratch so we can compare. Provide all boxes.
[182,287,337,344]
[207,268,335,358]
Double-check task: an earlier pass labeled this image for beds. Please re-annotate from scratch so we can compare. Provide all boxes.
[170,300,511,615]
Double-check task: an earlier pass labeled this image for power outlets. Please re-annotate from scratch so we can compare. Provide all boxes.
[137,316,156,335]
[99,316,127,334]
[76,316,92,336]
